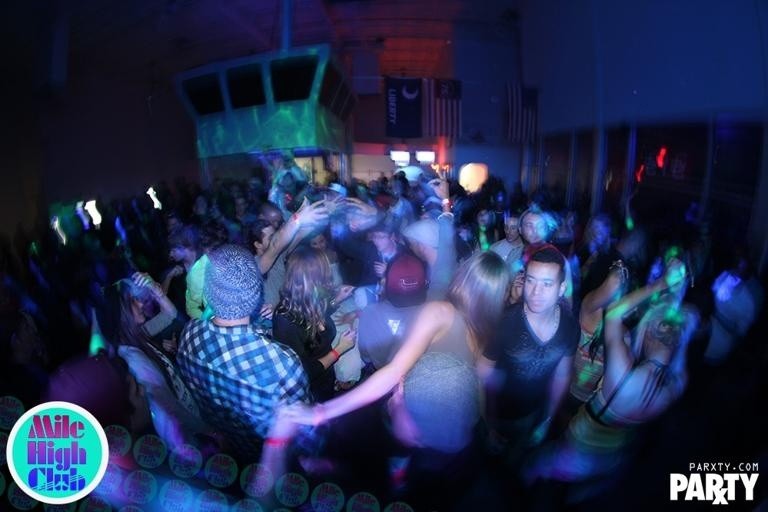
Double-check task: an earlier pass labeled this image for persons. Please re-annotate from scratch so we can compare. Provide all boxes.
[0,160,766,510]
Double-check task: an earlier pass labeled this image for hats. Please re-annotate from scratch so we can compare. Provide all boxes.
[386,253,427,295]
[200,241,266,321]
[259,202,283,229]
[398,217,442,247]
[42,352,125,418]
